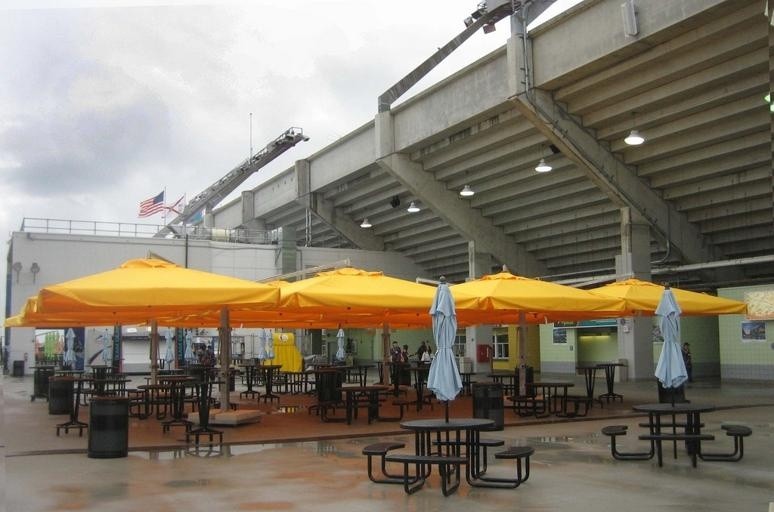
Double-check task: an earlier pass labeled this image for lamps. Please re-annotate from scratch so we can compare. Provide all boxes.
[534,144,552,173]
[622,111,644,146]
[406,202,421,214]
[31,262,40,284]
[13,262,22,284]
[460,171,475,197]
[359,207,372,229]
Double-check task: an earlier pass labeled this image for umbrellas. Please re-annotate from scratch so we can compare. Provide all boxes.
[653,283,688,459]
[425,276,464,484]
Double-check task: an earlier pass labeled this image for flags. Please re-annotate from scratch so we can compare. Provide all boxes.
[138,190,163,217]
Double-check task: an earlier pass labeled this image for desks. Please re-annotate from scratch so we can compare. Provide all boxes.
[632,402,716,454]
[400,418,496,475]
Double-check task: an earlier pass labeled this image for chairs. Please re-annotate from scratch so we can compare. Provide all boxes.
[32,361,627,441]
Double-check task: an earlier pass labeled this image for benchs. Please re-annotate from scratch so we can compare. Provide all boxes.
[433,438,504,456]
[720,423,752,462]
[361,441,405,484]
[385,453,469,497]
[638,422,706,445]
[494,446,536,492]
[604,425,629,458]
[641,434,714,466]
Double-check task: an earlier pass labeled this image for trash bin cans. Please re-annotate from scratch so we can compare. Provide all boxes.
[393,363,411,385]
[34,371,54,397]
[48,376,74,415]
[613,359,627,383]
[515,366,534,396]
[333,361,346,383]
[316,370,342,402]
[217,366,235,392]
[250,357,260,380]
[459,356,472,374]
[471,382,504,431]
[656,378,685,403]
[189,363,203,379]
[13,360,24,376]
[86,397,129,458]
